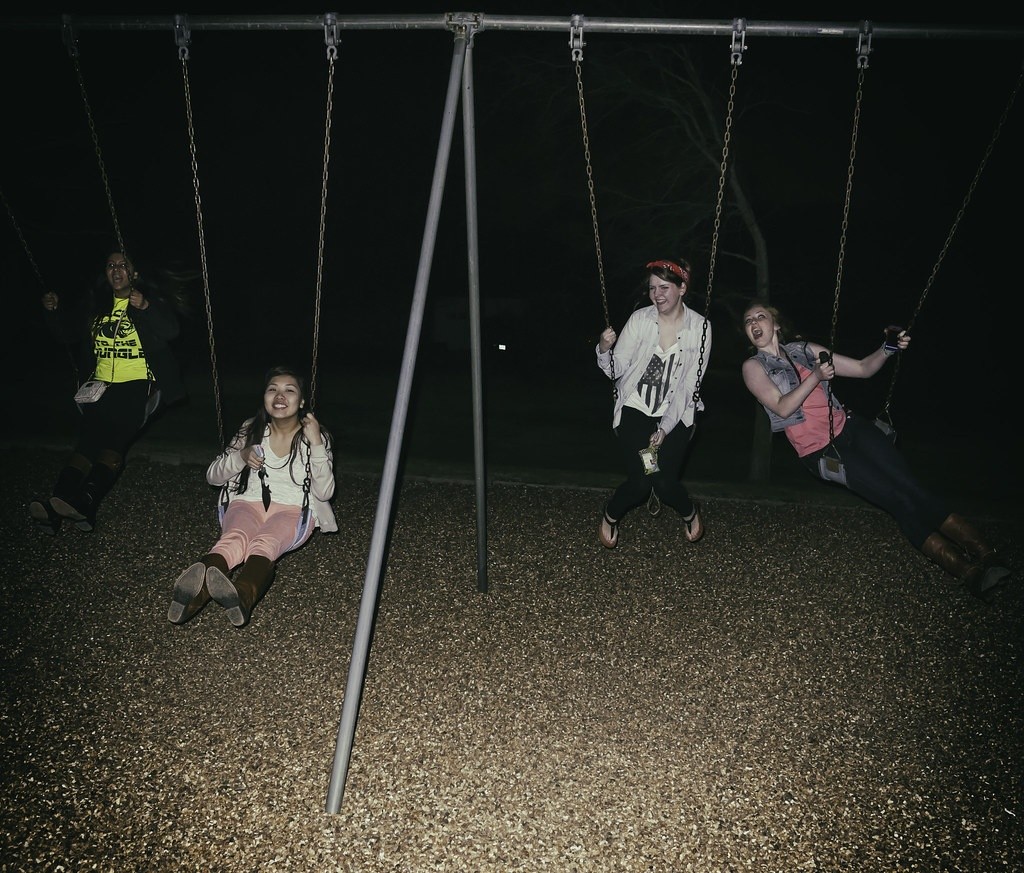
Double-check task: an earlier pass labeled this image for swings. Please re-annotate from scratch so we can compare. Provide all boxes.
[817,17,1024,492]
[567,14,750,443]
[171,9,343,555]
[0,14,163,434]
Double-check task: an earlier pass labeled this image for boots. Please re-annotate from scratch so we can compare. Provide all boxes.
[29,452,88,536]
[50,450,125,532]
[206,554,274,626]
[938,513,1009,585]
[922,531,995,602]
[167,553,230,624]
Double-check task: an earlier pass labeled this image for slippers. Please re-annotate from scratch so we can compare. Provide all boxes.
[600,500,617,548]
[683,504,703,542]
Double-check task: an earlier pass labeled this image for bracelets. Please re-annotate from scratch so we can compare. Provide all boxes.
[880,342,895,357]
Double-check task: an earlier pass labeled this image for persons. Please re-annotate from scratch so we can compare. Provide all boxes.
[166,365,340,628]
[594,258,712,548]
[28,249,159,532]
[739,298,1008,584]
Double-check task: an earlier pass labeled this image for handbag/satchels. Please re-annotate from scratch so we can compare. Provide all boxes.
[74,381,105,403]
